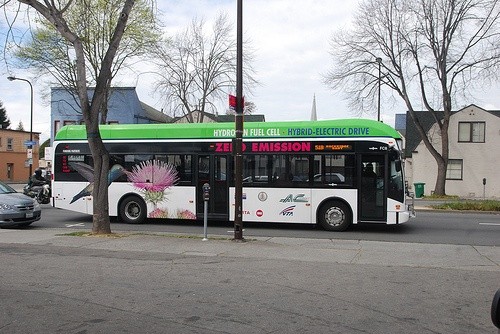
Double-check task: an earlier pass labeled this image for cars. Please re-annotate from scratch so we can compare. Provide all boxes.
[242,175,280,182]
[306,172,345,182]
[0,181,41,229]
[41,169,51,179]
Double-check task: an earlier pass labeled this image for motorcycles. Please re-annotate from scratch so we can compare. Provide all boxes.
[22,177,51,204]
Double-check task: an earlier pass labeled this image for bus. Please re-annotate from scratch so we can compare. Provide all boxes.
[49,118,417,232]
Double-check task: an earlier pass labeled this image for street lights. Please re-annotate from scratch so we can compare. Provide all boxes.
[375,57,383,120]
[8,76,33,179]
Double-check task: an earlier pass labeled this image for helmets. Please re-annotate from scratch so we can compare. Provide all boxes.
[35,169,42,175]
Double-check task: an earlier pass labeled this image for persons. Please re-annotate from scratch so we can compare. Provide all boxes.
[366,164,377,200]
[31,169,49,201]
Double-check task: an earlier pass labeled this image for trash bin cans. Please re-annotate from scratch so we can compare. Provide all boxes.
[414,182,425,198]
